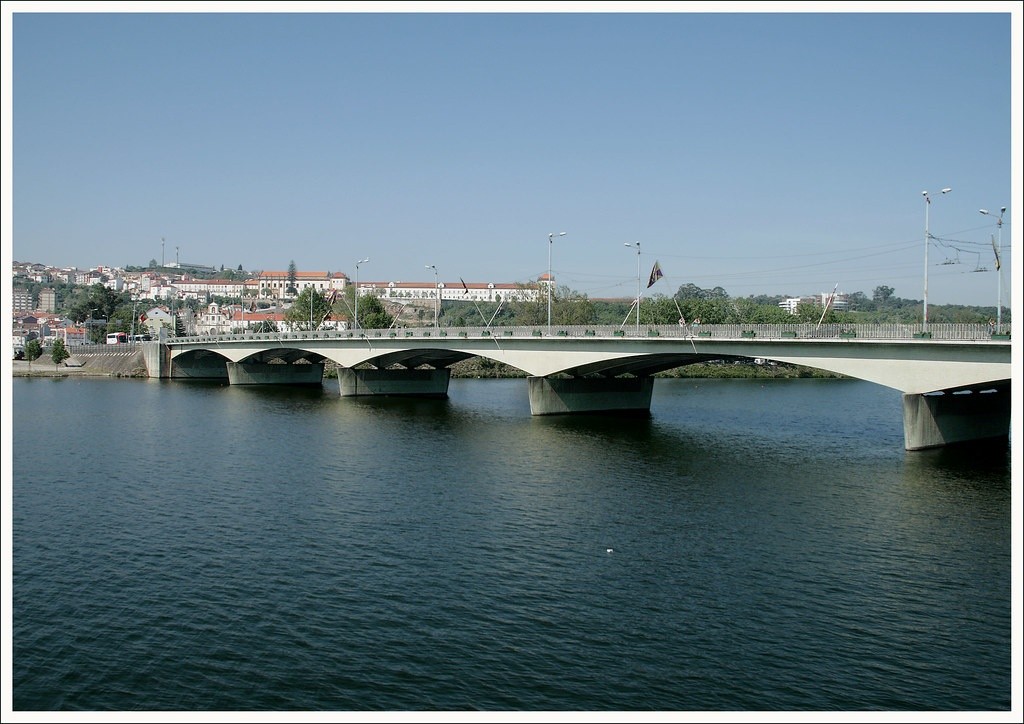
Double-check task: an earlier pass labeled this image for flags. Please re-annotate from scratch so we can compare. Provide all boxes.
[249,302,257,313]
[141,313,148,323]
[462,280,468,295]
[992,238,1000,271]
[647,262,663,288]
[327,289,336,305]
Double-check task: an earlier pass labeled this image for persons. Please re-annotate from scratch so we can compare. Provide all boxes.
[692,319,700,328]
[679,318,683,328]
[989,318,994,333]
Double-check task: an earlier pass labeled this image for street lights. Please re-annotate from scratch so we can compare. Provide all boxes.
[355,260,369,329]
[922,188,952,332]
[132,301,142,341]
[980,206,1006,334]
[425,265,438,328]
[624,241,641,334]
[546,232,567,337]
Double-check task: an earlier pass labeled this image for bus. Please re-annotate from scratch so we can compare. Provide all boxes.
[107,332,127,345]
[127,335,151,343]
